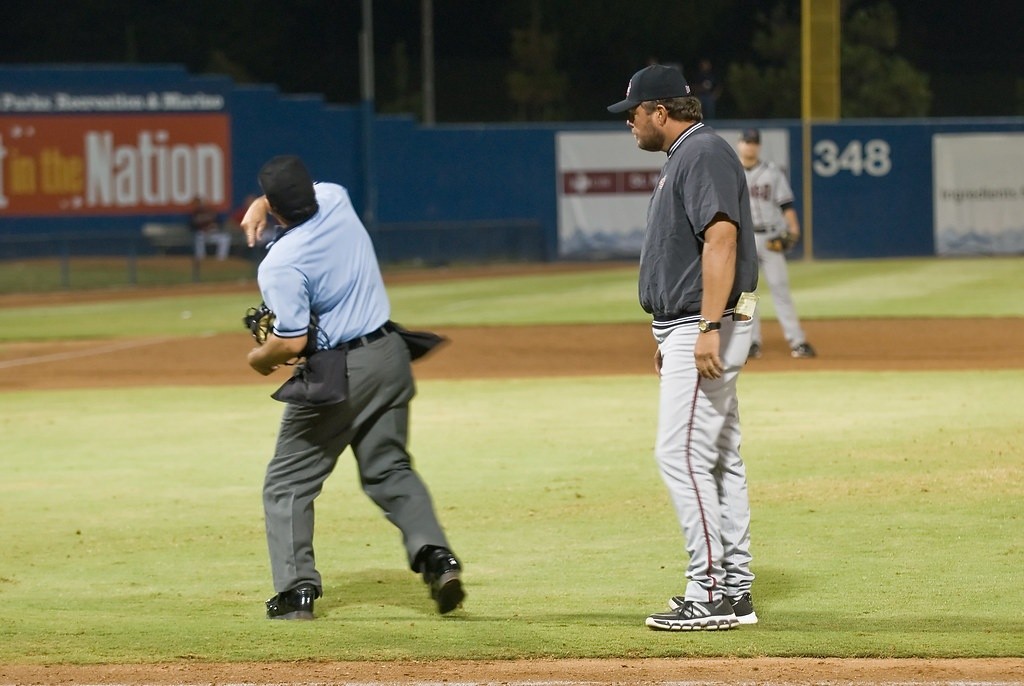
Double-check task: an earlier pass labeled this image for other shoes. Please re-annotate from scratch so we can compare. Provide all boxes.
[749,344,761,358]
[791,344,815,357]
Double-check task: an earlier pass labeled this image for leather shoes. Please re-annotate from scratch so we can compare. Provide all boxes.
[266,584,315,620]
[427,549,465,614]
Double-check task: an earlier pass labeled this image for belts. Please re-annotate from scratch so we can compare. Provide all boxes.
[348,322,395,350]
[754,228,775,232]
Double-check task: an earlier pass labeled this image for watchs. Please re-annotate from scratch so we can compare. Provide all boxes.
[699,317,721,333]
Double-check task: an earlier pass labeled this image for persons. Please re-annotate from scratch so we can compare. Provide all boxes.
[733,126,819,361]
[240,157,468,623]
[606,66,759,631]
[691,57,724,117]
[184,193,272,265]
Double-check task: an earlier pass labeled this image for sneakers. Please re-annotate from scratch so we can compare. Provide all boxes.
[646,597,740,630]
[669,592,758,624]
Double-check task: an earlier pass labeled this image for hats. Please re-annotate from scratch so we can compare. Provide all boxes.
[260,155,310,195]
[607,64,691,113]
[738,128,759,139]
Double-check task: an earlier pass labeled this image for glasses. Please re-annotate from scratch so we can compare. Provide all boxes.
[746,140,759,144]
